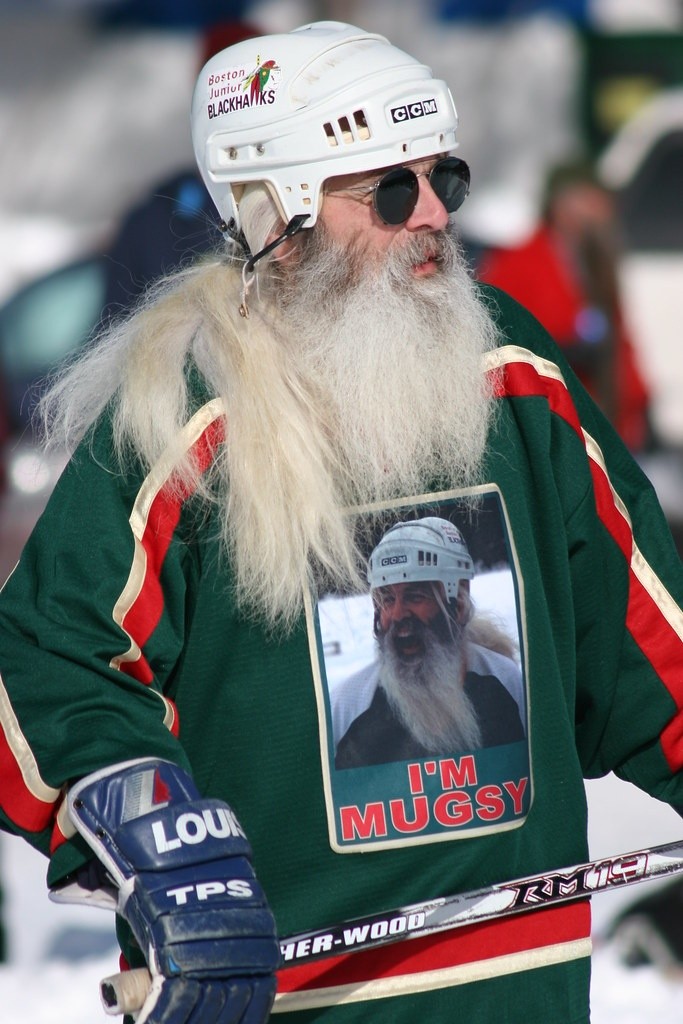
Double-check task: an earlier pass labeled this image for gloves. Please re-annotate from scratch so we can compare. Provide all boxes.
[68,757,281,1024]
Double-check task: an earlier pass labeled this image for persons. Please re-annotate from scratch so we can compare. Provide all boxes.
[329,518,525,770]
[103,20,270,336]
[0,20,683,1024]
[479,167,653,453]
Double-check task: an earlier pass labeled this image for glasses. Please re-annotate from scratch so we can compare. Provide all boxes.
[323,154,470,227]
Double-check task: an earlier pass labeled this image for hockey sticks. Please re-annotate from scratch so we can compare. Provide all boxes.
[101,836,682,1020]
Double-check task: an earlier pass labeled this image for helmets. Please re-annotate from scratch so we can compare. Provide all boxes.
[366,515,475,604]
[190,20,460,238]
